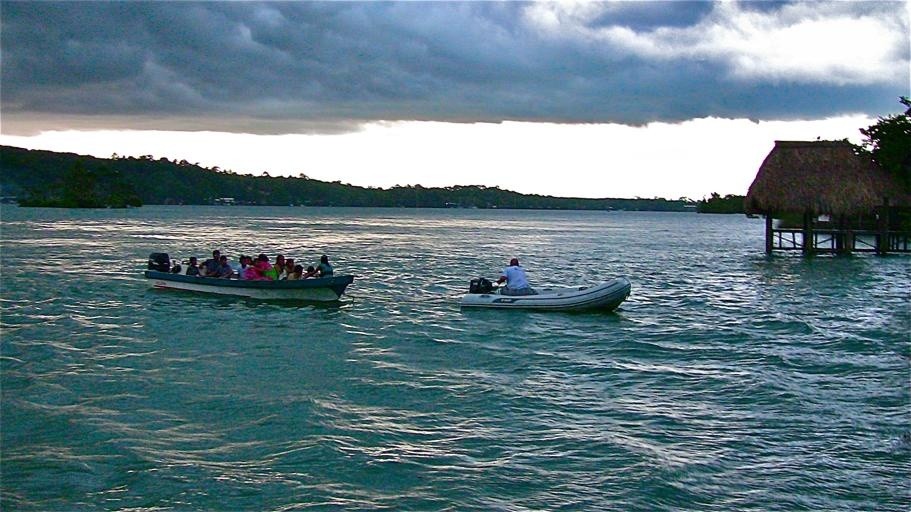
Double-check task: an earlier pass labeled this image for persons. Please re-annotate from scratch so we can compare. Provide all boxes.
[186,248,334,283]
[491,259,539,295]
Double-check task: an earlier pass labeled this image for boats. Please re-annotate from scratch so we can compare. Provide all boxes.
[146,287,344,330]
[145,251,355,301]
[460,278,632,312]
[462,307,620,328]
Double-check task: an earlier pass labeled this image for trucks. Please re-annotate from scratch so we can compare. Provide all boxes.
[445,203,456,208]
[215,198,235,204]
